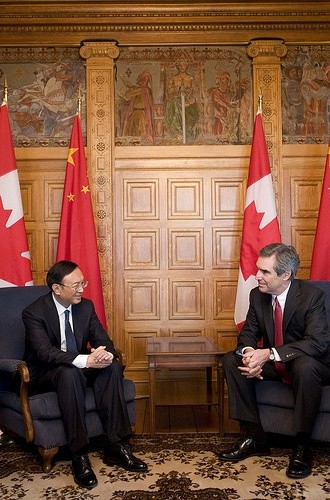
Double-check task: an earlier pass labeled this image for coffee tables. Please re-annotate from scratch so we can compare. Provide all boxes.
[144,351,227,439]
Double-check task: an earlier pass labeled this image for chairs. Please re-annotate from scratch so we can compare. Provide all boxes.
[0,285,136,473]
[255,281,330,443]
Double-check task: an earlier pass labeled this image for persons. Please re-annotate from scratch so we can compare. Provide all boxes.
[19,261,147,488]
[219,243,330,479]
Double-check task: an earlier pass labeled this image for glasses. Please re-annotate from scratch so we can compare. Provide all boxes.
[56,281,88,290]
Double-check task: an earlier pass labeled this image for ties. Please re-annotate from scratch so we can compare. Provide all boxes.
[64,311,78,355]
[274,297,292,384]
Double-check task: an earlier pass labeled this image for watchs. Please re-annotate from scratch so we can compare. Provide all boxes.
[269,349,274,362]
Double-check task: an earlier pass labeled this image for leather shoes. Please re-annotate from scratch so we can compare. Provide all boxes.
[72,456,97,487]
[219,438,271,461]
[287,449,311,477]
[103,448,147,471]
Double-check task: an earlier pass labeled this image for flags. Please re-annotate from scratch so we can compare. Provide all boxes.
[310,140,330,281]
[0,98,34,288]
[234,109,281,348]
[55,112,108,348]
